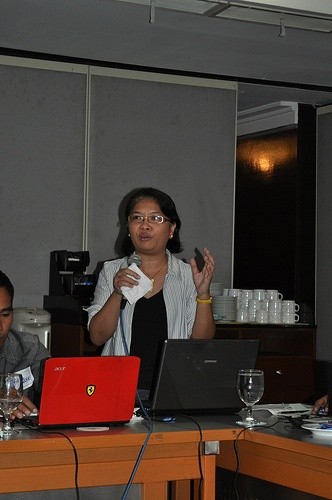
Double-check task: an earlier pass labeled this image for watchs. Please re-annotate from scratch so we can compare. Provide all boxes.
[194,295,214,304]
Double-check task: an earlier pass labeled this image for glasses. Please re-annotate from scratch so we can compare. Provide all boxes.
[127,214,173,225]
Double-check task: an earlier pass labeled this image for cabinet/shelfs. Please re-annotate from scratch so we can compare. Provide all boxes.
[215,324,316,396]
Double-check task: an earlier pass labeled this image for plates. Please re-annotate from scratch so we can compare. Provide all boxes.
[209,283,224,296]
[212,296,237,321]
[301,423,332,436]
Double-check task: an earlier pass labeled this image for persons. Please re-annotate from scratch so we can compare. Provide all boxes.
[311,391,332,415]
[0,270,52,420]
[82,187,216,390]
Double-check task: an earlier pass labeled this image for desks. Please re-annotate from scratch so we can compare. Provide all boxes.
[0,409,332,500]
[43,294,101,357]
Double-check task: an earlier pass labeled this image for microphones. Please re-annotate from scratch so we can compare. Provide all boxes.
[120,254,141,311]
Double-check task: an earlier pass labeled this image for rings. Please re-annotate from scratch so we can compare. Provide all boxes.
[212,272,215,276]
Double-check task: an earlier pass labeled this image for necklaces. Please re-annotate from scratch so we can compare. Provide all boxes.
[139,266,164,292]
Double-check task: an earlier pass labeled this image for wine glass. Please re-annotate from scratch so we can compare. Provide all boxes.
[0,373,23,436]
[236,369,264,427]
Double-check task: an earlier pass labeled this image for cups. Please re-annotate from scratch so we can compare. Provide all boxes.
[224,289,299,324]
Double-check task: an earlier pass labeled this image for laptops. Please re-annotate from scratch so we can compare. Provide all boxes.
[135,339,262,416]
[15,355,140,430]
